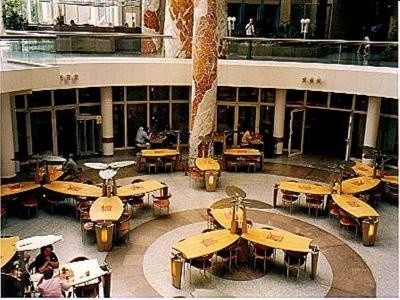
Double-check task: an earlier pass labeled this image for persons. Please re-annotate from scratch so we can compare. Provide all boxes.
[134,126,153,144]
[245,18,256,59]
[69,20,78,27]
[34,269,70,298]
[241,129,253,147]
[363,35,371,58]
[56,157,77,180]
[34,244,59,272]
[1,262,24,298]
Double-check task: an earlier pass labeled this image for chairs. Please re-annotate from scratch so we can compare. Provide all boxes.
[151,192,172,216]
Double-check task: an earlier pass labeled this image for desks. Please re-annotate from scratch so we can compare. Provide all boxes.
[224,148,264,171]
[169,208,319,289]
[0,164,168,271]
[196,157,220,192]
[24,259,112,298]
[273,161,399,241]
[136,149,181,172]
